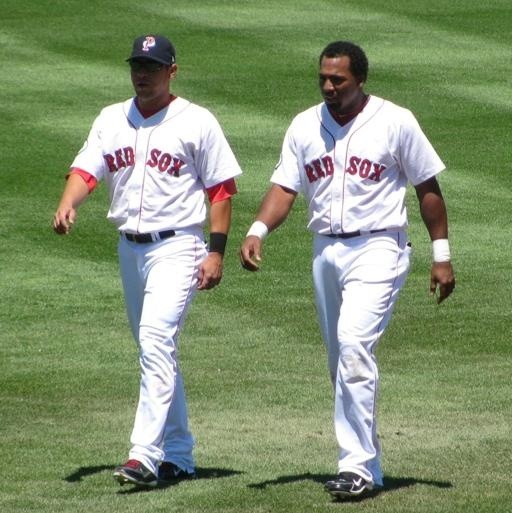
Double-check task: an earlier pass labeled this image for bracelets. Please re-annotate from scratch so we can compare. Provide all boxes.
[246,219,269,241]
[432,238,452,262]
[210,233,227,255]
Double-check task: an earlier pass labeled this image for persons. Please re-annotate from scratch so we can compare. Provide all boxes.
[53,34,244,490]
[240,41,454,498]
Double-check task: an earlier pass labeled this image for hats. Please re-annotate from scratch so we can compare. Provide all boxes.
[124,33,177,69]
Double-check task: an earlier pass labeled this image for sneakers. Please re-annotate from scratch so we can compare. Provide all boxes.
[113,459,159,488]
[159,461,197,484]
[323,472,382,499]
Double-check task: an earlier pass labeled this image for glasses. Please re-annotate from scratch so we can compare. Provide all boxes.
[128,61,168,72]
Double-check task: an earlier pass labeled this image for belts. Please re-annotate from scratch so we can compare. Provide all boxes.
[117,230,175,245]
[320,227,386,241]
